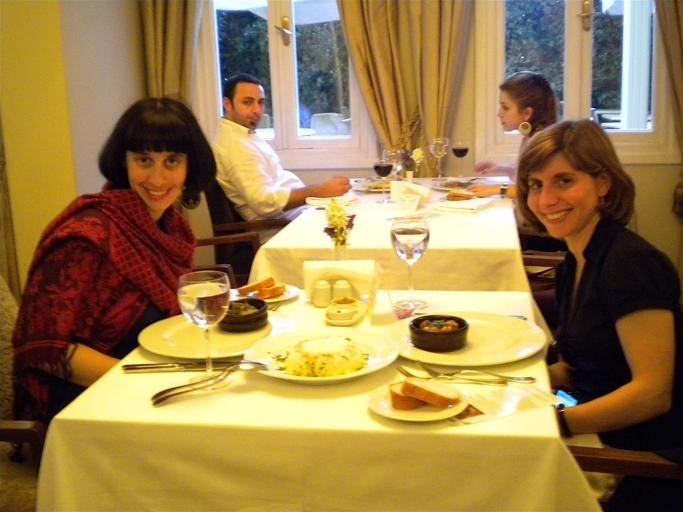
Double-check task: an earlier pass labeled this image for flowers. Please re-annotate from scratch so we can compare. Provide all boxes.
[321,195,356,253]
[401,146,425,173]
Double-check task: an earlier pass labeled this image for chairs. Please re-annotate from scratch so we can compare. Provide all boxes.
[190,232,259,288]
[0,274,60,512]
[516,232,569,309]
[252,110,352,143]
[568,444,682,512]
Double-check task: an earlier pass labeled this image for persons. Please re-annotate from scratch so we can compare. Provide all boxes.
[212,73,350,244]
[513,118,683,512]
[468,71,566,252]
[13,94,216,465]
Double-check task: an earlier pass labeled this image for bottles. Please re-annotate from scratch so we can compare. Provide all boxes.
[335,280,353,299]
[313,279,333,308]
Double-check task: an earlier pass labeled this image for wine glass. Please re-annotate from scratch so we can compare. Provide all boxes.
[176,269,231,379]
[373,135,470,208]
[385,219,429,311]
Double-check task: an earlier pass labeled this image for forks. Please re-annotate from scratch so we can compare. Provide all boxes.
[152,359,264,409]
[394,360,539,387]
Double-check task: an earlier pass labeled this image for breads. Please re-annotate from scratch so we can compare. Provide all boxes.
[446,190,476,201]
[236,276,275,295]
[389,381,427,410]
[401,377,458,409]
[258,282,286,300]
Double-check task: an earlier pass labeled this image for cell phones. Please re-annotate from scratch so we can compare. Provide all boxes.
[554,387,581,410]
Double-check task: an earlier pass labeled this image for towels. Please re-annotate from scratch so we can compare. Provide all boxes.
[304,189,359,209]
[388,180,431,206]
[434,197,495,213]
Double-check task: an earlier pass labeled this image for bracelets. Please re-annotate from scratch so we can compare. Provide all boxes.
[557,403,571,439]
[501,183,507,198]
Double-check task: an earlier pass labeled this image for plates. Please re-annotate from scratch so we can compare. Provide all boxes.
[392,312,549,366]
[367,383,469,423]
[243,329,399,385]
[138,312,272,361]
[324,302,366,326]
[233,284,300,303]
[350,183,391,193]
[221,298,267,329]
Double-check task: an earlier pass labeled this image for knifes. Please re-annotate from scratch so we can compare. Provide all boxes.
[121,361,236,374]
